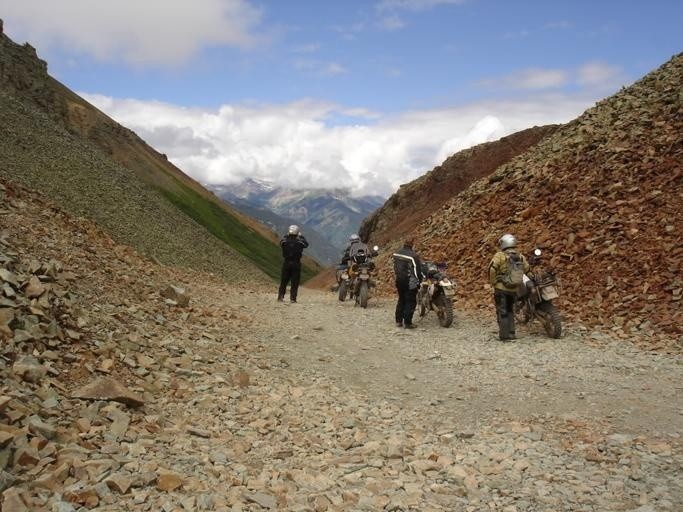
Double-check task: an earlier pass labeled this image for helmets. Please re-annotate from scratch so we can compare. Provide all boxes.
[349,233,360,243]
[288,224,300,236]
[498,233,517,251]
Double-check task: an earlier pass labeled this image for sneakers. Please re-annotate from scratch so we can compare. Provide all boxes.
[405,324,417,328]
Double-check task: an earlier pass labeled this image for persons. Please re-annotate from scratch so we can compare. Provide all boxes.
[486,234,529,340]
[276,224,308,303]
[392,238,423,328]
[340,233,377,264]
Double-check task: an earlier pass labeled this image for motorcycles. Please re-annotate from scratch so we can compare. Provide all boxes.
[416,261,456,328]
[332,245,381,309]
[512,248,562,338]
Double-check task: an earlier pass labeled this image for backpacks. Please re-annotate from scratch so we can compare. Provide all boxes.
[497,252,524,289]
